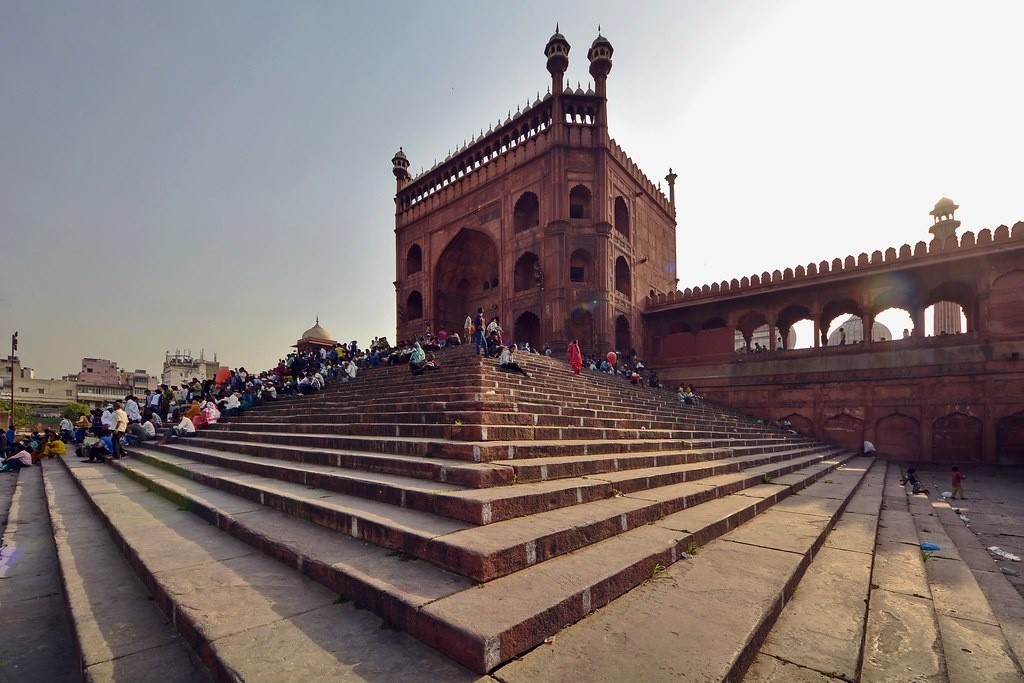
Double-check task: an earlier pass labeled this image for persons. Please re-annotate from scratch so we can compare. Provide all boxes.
[951,465,969,500]
[899,468,930,494]
[742,322,961,355]
[783,418,792,430]
[5,333,358,472]
[362,303,553,379]
[567,336,705,406]
